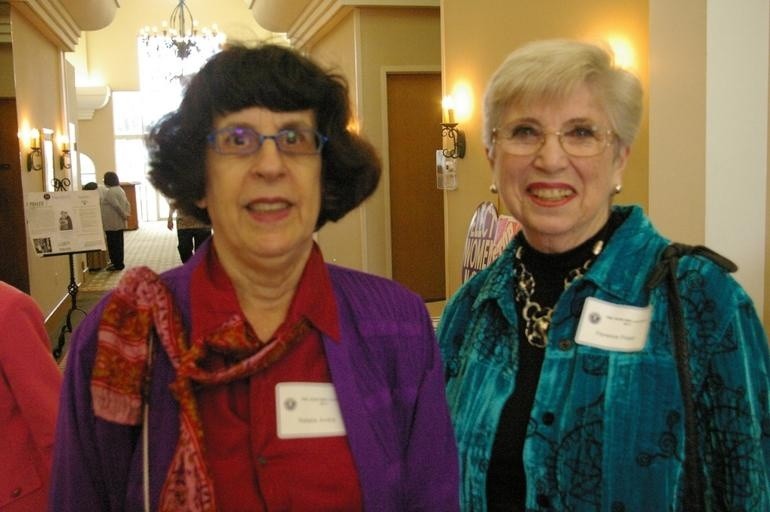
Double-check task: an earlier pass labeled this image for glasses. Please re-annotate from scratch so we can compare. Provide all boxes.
[491,120,619,157]
[204,126,328,156]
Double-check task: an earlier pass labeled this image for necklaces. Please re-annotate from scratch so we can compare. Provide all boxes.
[512,217,610,349]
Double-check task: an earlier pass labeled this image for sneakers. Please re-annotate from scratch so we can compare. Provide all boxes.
[106,264,124,271]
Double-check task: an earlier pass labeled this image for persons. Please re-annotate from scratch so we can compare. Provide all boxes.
[63,211,73,230]
[0,281,63,512]
[99,170,132,272]
[84,181,98,190]
[167,202,212,265]
[59,210,68,230]
[43,43,460,512]
[434,38,770,512]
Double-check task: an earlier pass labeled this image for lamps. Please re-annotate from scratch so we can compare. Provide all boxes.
[20,121,42,172]
[59,134,72,169]
[137,0,225,88]
[440,82,475,161]
